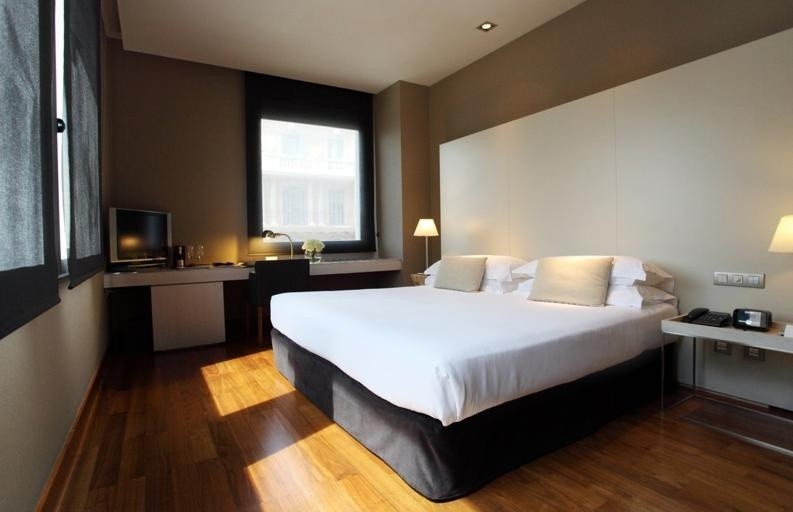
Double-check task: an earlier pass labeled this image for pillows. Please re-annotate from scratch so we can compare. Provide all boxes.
[424,253,679,312]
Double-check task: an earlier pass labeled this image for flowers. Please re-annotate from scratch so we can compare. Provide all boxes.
[300,239,324,252]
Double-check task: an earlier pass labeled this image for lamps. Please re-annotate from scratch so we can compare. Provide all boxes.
[262,230,293,259]
[413,217,439,275]
[766,215,793,338]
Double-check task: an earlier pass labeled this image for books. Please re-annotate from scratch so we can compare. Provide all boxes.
[195,263,211,269]
[783,323,793,339]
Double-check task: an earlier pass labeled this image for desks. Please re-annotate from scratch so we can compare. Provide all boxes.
[103,253,400,360]
[658,308,792,459]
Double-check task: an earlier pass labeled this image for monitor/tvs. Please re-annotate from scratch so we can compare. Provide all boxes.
[103,207,174,272]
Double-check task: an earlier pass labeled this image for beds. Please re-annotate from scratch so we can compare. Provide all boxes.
[267,249,679,501]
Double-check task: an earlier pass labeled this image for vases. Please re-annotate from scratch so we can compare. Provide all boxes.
[304,250,321,264]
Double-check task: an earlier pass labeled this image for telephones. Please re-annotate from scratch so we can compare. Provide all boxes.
[682,307,731,327]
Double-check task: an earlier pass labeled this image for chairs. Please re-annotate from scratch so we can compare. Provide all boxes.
[247,258,312,347]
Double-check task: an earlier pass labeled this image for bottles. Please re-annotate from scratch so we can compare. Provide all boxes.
[175,246,182,267]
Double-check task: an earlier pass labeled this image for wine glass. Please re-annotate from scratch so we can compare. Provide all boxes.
[186,245,193,266]
[196,244,203,266]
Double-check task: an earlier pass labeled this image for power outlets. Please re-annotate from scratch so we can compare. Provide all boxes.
[743,345,765,361]
[712,341,731,356]
